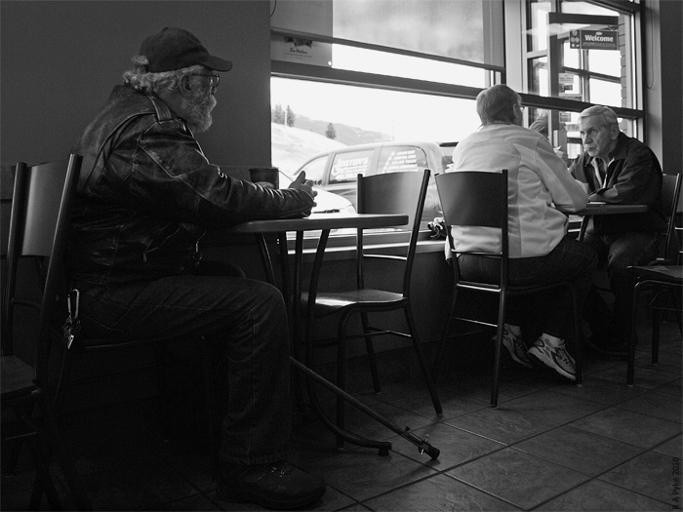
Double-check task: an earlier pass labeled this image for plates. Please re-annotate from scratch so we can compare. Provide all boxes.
[585,200,607,206]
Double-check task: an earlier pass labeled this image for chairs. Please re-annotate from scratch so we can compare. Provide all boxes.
[431,170,581,408]
[1,154,90,511]
[291,167,442,425]
[577,171,683,385]
[9,257,217,511]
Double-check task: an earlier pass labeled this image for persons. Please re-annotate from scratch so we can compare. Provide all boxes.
[568,103,665,370]
[442,84,588,386]
[59,24,326,510]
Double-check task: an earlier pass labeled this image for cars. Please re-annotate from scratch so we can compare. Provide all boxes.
[269,160,359,239]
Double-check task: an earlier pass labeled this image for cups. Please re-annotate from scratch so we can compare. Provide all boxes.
[248,167,280,191]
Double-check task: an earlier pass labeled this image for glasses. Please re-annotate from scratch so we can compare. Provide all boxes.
[192,73,219,85]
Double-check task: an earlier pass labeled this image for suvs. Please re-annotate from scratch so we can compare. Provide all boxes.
[292,136,462,224]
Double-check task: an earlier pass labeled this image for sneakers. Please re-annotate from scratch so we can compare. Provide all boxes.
[215,468,326,508]
[529,332,578,381]
[495,324,530,369]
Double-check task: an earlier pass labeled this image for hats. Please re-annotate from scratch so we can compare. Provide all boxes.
[139,26,232,73]
